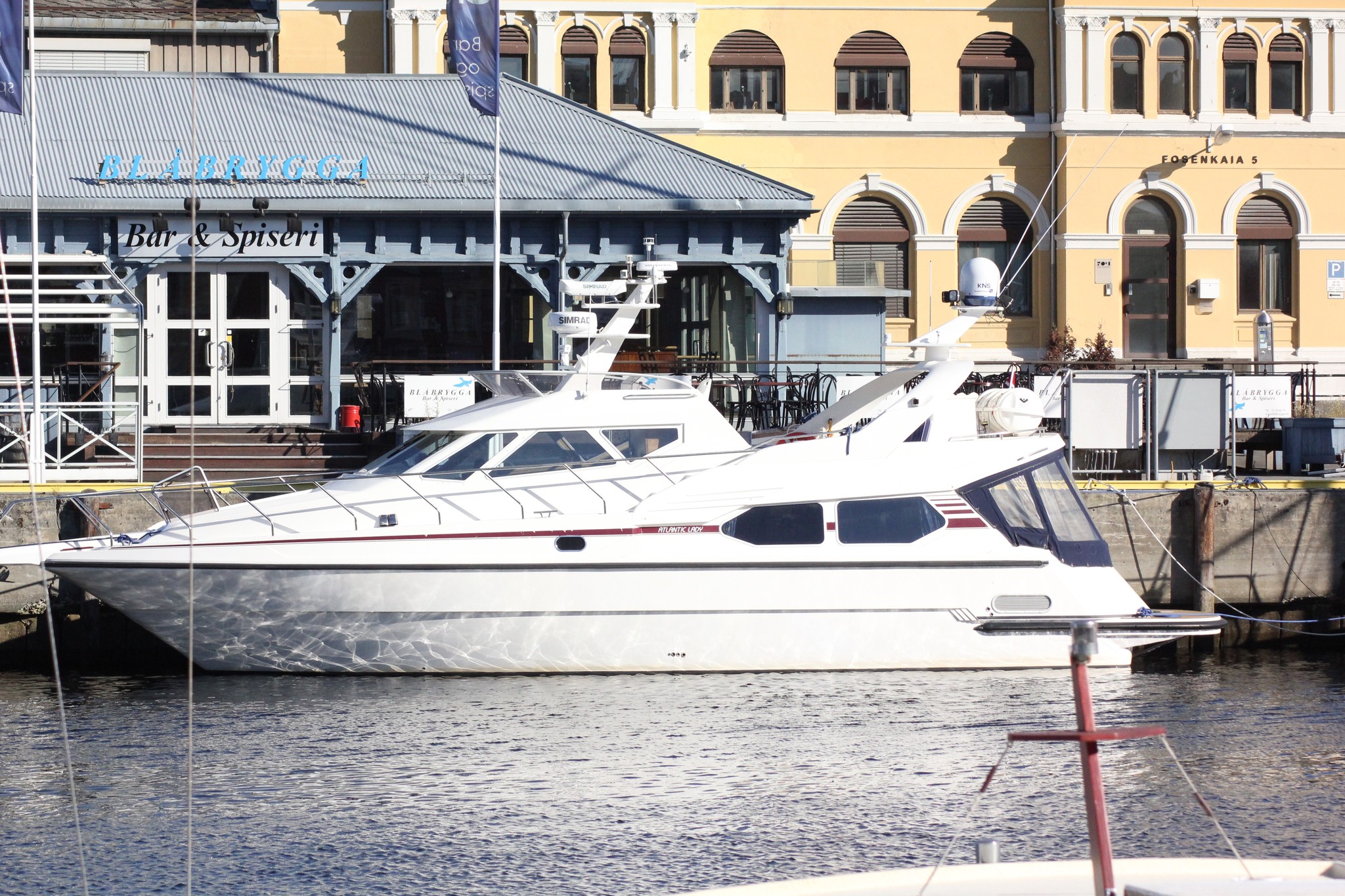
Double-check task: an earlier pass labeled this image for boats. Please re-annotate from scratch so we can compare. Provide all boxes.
[669,624,1345,896]
[24,152,1230,676]
[0,536,142,620]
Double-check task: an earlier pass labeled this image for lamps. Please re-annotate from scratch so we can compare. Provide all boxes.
[100,352,114,375]
[286,212,303,233]
[183,197,200,210]
[219,213,235,231]
[942,291,965,306]
[253,197,269,215]
[776,292,795,320]
[330,291,342,319]
[620,270,628,280]
[1000,295,1014,309]
[152,213,169,231]
[572,295,582,305]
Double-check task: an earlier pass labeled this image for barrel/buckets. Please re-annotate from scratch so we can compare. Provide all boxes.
[340,405,360,427]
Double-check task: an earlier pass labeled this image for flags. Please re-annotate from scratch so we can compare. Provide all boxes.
[0,0,25,117]
[446,0,500,118]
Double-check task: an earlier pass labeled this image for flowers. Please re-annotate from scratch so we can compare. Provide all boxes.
[1292,401,1345,419]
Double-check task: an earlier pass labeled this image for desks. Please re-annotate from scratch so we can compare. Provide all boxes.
[963,382,993,394]
[756,382,803,429]
[713,383,747,417]
[0,384,60,446]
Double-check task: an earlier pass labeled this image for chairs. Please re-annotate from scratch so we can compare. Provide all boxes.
[634,346,1301,478]
[370,374,425,433]
[0,414,28,464]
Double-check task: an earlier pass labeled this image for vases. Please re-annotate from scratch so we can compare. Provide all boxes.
[1280,418,1345,477]
